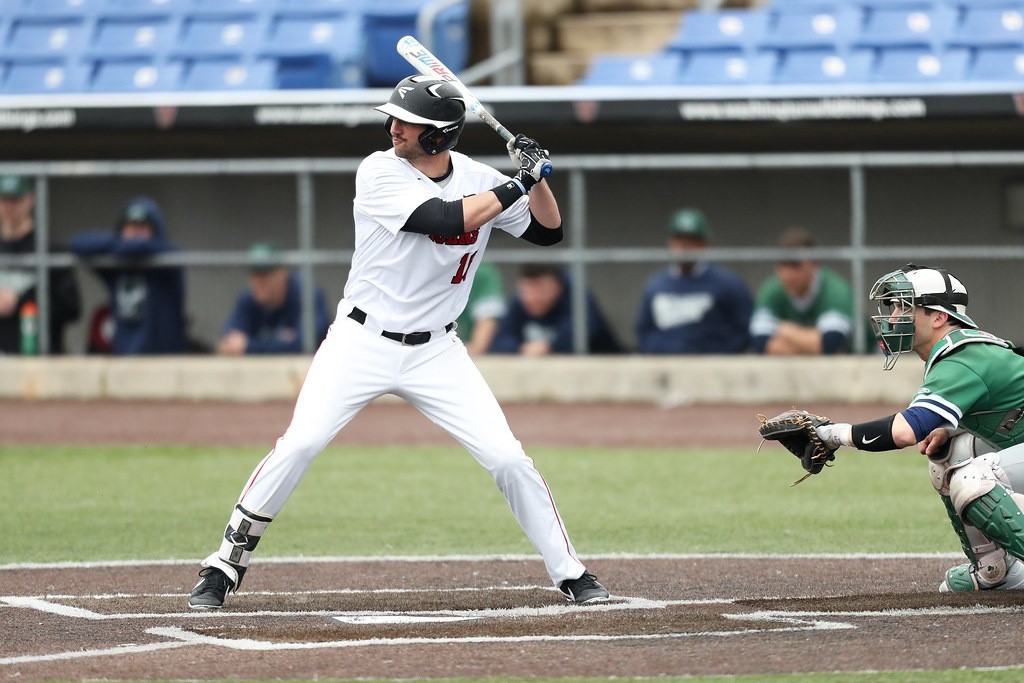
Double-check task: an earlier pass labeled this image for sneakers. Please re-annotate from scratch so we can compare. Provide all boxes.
[559,566,611,606]
[188,566,233,609]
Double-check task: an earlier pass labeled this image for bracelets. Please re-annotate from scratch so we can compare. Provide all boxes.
[511,178,526,195]
[850,413,905,453]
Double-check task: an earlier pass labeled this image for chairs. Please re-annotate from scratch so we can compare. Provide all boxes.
[0,0,469,96]
[566,0,1024,85]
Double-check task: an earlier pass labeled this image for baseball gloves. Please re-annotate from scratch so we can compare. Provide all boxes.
[757,408,842,475]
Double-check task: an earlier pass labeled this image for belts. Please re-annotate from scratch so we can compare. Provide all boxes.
[348,308,455,346]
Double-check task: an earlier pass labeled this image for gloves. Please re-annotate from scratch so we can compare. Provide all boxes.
[505,133,543,172]
[513,142,555,197]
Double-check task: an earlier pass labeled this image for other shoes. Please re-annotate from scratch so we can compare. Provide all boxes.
[939,558,1024,593]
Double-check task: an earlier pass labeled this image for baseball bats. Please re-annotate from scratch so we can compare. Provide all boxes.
[396,35,554,178]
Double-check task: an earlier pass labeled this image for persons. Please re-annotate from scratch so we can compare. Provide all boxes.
[455,263,628,354]
[214,240,332,354]
[0,173,84,355]
[189,73,609,611]
[756,266,1024,592]
[632,205,876,356]
[73,198,191,354]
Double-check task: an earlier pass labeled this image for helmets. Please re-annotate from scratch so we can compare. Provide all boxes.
[373,74,467,158]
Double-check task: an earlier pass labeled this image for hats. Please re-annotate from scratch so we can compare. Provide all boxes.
[120,194,166,235]
[671,207,712,242]
[247,243,282,275]
[0,173,32,199]
[880,268,981,329]
[773,230,819,259]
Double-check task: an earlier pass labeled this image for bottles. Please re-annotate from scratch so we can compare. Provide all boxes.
[20,304,40,355]
